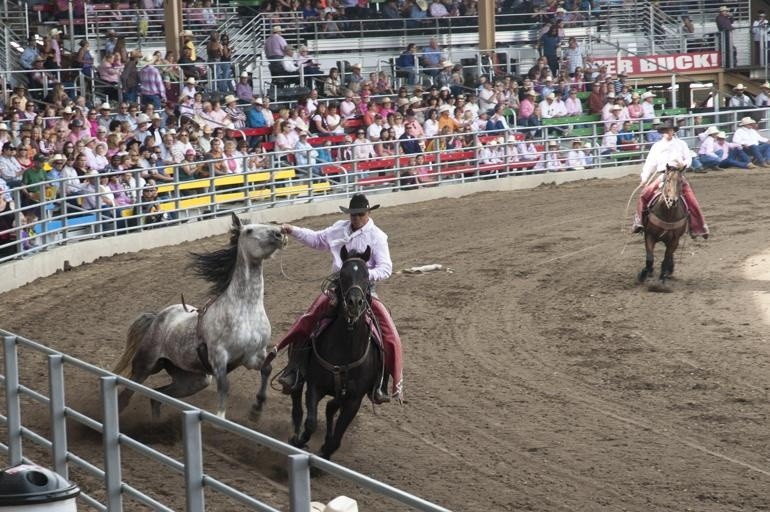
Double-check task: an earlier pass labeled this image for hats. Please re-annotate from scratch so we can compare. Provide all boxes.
[68,120,82,129]
[35,152,68,166]
[738,117,756,126]
[733,83,747,89]
[760,83,770,89]
[33,55,46,61]
[182,78,197,86]
[251,98,264,105]
[136,113,162,124]
[49,29,62,37]
[238,70,249,78]
[416,1,428,10]
[225,94,240,105]
[549,141,559,147]
[382,85,424,107]
[202,126,212,134]
[571,139,591,148]
[706,125,729,139]
[489,136,516,144]
[187,149,193,155]
[182,30,192,36]
[607,91,656,111]
[128,50,156,66]
[339,194,379,215]
[653,118,680,133]
[273,25,284,32]
[476,108,489,117]
[555,8,566,13]
[13,84,27,94]
[126,139,142,152]
[439,106,449,112]
[97,103,113,111]
[59,106,75,114]
[719,6,729,12]
[0,124,15,150]
[85,136,108,156]
[526,77,555,101]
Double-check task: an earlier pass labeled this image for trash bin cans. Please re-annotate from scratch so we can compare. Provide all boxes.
[0,465,80,512]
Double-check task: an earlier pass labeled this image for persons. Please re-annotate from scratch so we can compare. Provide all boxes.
[278,193,393,404]
[0,0,770,265]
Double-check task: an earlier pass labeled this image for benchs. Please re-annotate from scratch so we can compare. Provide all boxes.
[1,1,730,237]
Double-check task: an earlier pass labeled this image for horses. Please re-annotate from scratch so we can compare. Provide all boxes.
[105,210,290,435]
[288,244,385,481]
[636,163,691,286]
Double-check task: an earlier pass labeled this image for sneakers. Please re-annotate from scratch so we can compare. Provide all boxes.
[280,371,304,399]
[374,390,391,404]
